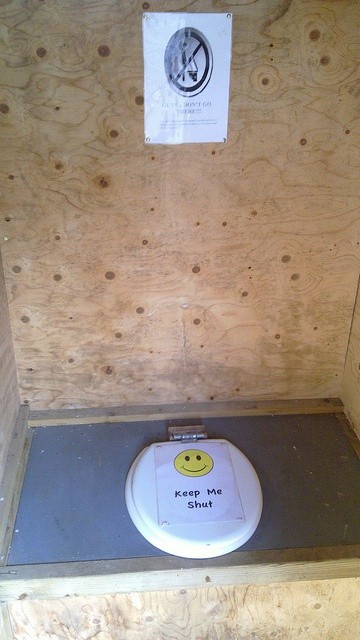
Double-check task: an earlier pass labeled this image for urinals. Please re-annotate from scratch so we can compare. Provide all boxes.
[124,424,264,559]
